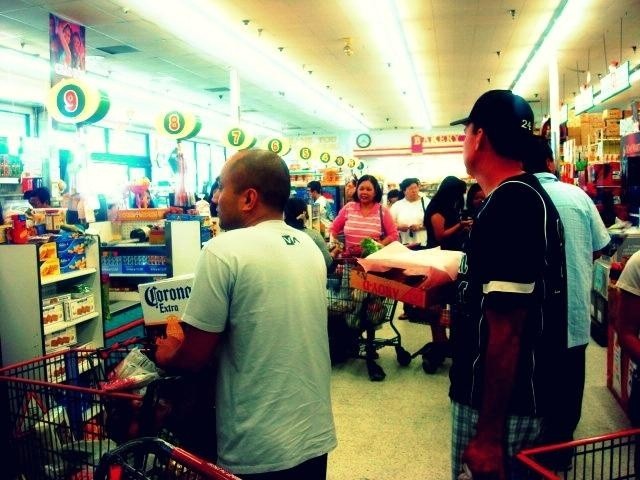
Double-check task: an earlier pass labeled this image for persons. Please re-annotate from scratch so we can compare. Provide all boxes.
[528,135,616,472]
[133,174,485,322]
[29,189,52,208]
[177,149,337,480]
[48,15,88,71]
[616,249,640,363]
[447,89,567,480]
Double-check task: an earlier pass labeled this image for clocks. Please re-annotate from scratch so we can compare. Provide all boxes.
[356,133,371,149]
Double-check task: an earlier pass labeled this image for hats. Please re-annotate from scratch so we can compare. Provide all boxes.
[449,88,536,137]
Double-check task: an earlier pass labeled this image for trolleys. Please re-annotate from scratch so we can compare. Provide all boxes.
[325,257,411,382]
[65,438,241,480]
[513,427,640,479]
[0,347,187,480]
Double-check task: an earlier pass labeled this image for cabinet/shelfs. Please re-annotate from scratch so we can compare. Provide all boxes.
[85,219,200,340]
[0,231,103,426]
